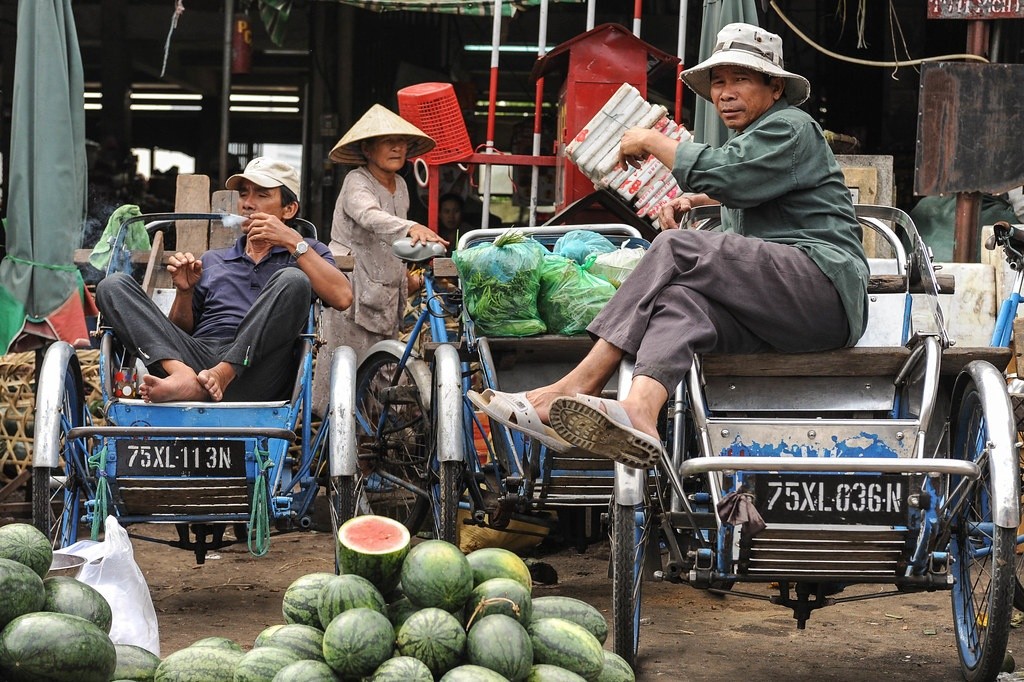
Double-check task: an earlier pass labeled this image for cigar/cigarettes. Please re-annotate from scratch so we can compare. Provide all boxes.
[228,212,248,220]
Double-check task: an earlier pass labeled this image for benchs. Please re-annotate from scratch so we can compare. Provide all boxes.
[457,224,643,369]
[701,272,1013,375]
[100,213,319,408]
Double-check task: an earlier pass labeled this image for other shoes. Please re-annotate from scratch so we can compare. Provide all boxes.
[74,261,106,285]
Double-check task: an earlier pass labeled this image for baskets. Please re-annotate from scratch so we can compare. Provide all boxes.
[397,81,474,165]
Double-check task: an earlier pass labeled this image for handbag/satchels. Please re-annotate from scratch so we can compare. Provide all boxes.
[452,229,646,338]
[51,514,160,660]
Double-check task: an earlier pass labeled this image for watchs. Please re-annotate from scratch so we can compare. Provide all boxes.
[291,240,310,259]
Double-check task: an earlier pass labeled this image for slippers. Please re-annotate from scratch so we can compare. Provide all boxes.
[547,393,658,470]
[362,471,393,492]
[467,388,572,455]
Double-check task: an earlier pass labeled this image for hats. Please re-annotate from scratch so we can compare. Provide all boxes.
[678,22,810,107]
[327,103,437,164]
[225,156,301,202]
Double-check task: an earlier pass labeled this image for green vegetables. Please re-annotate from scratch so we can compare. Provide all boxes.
[450,223,621,336]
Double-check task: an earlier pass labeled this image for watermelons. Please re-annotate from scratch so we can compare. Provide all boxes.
[1,514,635,682]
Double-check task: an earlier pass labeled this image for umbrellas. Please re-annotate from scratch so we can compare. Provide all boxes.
[693,1,758,230]
[1,0,99,350]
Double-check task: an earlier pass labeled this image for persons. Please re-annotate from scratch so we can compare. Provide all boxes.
[310,103,450,423]
[439,196,474,250]
[93,157,352,411]
[460,20,870,469]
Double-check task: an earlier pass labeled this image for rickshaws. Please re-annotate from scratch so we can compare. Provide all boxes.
[356,188,1023,682]
[30,214,361,572]
